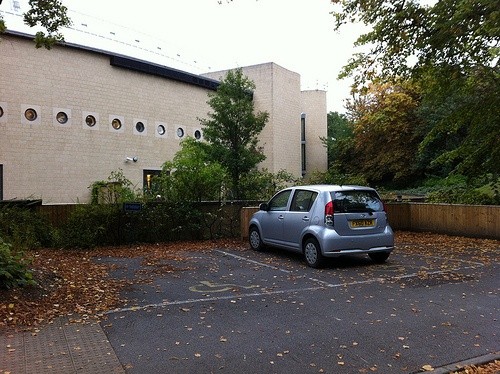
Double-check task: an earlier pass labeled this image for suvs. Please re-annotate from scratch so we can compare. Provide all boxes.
[247,184,395,267]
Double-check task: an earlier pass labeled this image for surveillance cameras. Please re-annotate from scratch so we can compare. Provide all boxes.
[126,157,133,162]
